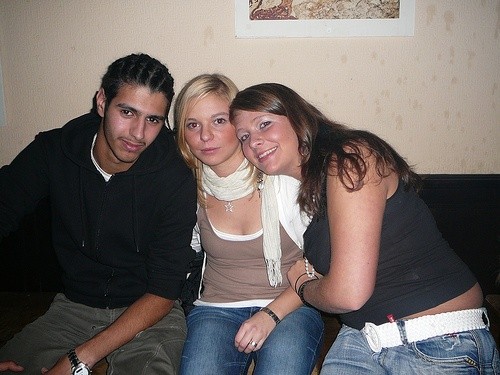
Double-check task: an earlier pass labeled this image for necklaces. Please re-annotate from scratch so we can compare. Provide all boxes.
[304,193,315,277]
[225,201,233,212]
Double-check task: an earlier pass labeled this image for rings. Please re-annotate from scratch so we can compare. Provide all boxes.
[250,341,257,347]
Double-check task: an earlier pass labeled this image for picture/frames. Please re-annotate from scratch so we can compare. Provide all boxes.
[234,0,414,37]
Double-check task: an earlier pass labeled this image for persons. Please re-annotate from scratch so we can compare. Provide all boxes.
[0,53,198,375]
[174,73,326,375]
[229,83,500,375]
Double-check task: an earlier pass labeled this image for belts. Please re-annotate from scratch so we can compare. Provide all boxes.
[362,309,486,352]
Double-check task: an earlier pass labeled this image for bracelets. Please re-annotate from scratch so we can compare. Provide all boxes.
[298,279,318,307]
[261,308,281,325]
[295,272,319,294]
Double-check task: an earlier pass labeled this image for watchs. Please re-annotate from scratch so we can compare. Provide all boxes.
[67,346,92,375]
